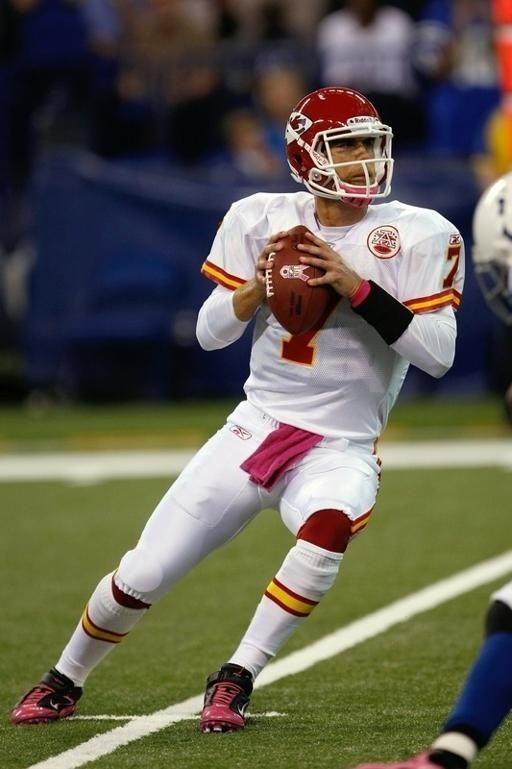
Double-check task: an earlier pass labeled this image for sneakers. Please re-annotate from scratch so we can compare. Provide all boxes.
[200,664,251,731]
[355,747,469,769]
[12,665,83,722]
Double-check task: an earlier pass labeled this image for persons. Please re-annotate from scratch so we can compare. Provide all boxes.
[12,84,464,735]
[1,2,511,187]
[348,172,510,769]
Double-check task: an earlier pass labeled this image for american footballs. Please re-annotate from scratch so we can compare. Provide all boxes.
[264,226,328,336]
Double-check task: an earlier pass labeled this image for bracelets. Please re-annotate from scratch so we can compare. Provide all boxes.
[350,279,415,346]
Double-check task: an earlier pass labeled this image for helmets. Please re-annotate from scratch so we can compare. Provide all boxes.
[471,172,511,326]
[285,87,393,200]
